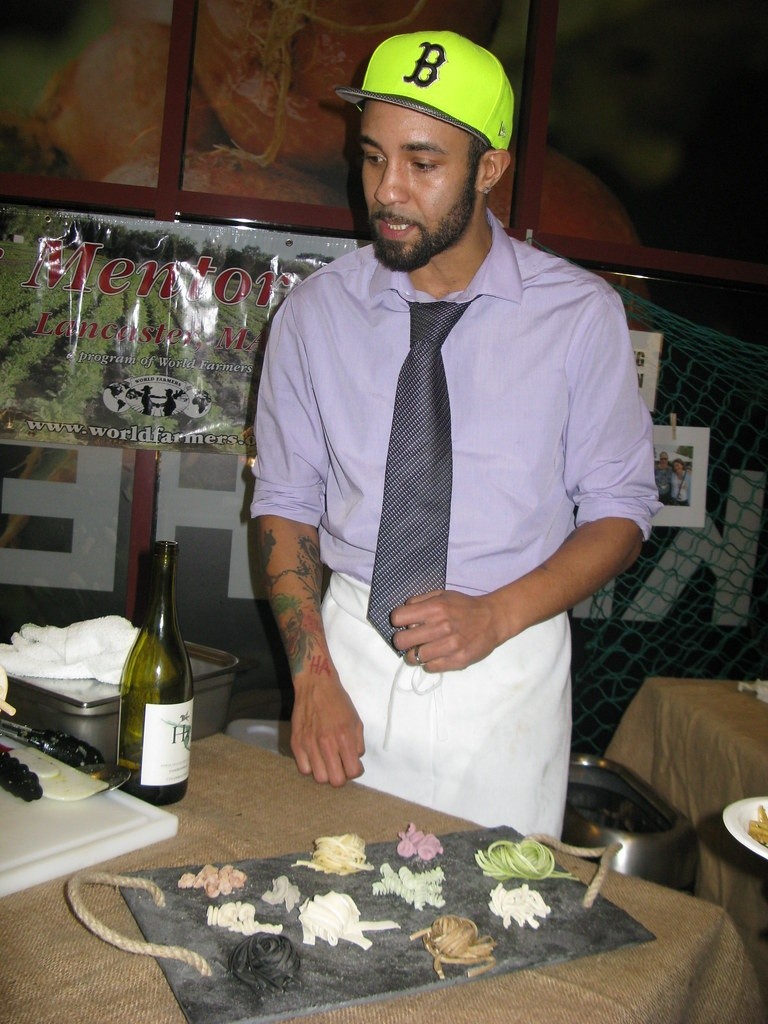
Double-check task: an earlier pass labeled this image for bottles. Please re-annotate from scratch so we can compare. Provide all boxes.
[115,541,195,806]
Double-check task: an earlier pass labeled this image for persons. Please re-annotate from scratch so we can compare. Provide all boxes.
[654,451,691,506]
[243,30,664,847]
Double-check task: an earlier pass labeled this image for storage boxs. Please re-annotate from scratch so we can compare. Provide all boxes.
[0,640,240,759]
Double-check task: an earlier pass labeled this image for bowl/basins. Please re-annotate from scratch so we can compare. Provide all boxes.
[7,631,240,762]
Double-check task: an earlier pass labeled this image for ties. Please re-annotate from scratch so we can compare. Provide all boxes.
[366,300,473,658]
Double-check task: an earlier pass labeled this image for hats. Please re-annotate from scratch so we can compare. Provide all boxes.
[334,31,514,151]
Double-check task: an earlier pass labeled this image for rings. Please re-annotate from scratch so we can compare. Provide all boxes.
[415,647,426,666]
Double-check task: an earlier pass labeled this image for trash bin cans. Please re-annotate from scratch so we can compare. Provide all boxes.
[560,752,700,895]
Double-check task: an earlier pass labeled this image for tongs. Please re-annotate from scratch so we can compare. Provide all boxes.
[0,719,104,803]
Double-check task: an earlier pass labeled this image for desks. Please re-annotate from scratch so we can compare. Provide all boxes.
[0,742,768,1024]
[604,675,768,996]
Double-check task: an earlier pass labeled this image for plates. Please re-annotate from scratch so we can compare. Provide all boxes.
[722,796,768,861]
